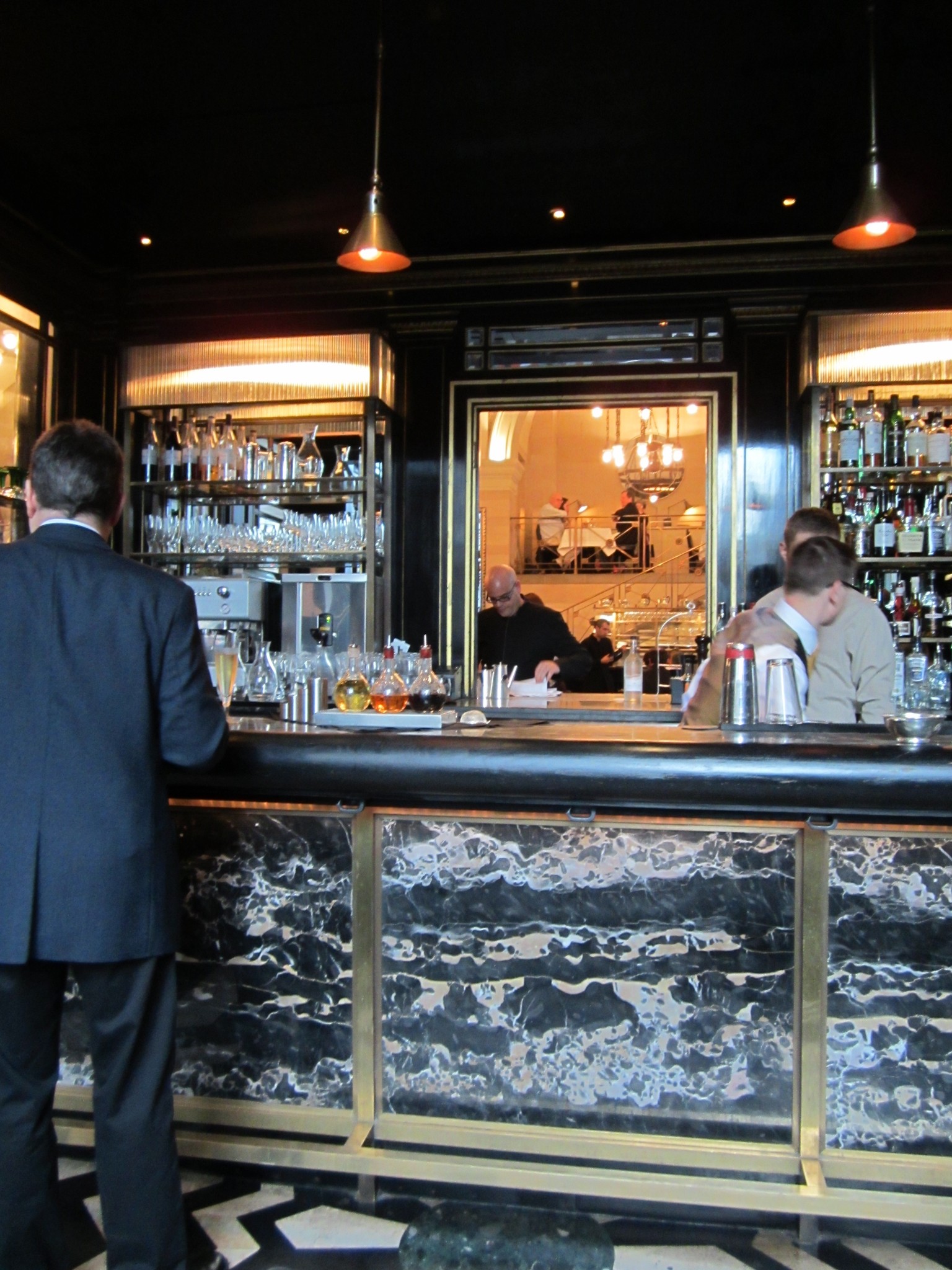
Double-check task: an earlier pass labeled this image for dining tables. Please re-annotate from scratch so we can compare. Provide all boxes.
[558,527,617,573]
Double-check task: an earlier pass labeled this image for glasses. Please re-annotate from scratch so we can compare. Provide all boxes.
[485,582,515,603]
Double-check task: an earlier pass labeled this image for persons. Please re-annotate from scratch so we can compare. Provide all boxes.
[576,616,621,690]
[753,506,898,729]
[0,419,236,1270]
[476,559,587,686]
[665,534,857,728]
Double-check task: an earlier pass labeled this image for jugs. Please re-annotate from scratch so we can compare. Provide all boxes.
[481,660,518,702]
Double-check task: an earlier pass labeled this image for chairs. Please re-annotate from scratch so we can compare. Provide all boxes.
[686,527,706,573]
[616,514,649,574]
[535,524,566,574]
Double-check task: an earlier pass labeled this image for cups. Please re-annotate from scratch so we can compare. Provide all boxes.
[764,657,804,727]
[721,642,758,727]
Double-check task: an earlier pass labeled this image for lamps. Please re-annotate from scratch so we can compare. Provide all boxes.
[830,1,916,252]
[334,1,411,272]
[600,406,685,469]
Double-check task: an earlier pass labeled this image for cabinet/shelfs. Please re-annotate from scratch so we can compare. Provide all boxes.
[116,393,393,651]
[795,379,952,671]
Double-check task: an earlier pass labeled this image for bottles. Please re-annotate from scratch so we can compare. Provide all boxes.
[889,621,906,714]
[821,386,952,640]
[623,636,642,701]
[139,408,447,721]
[906,636,925,713]
[928,642,950,719]
[684,599,759,690]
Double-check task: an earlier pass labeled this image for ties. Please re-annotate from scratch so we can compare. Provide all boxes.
[807,649,817,675]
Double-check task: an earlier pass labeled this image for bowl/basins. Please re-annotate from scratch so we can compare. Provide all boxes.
[884,713,945,745]
